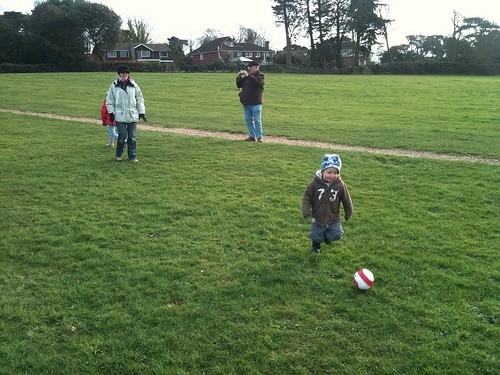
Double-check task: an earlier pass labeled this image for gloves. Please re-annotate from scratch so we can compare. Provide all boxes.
[109,112,115,122]
[139,113,148,122]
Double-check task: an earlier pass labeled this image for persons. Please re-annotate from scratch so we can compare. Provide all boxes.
[236,61,265,142]
[105,65,147,161]
[101,99,127,148]
[301,154,353,253]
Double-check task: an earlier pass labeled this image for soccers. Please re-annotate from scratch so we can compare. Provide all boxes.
[353,268,375,291]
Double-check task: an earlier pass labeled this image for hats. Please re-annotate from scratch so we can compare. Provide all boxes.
[321,153,341,172]
[248,60,260,65]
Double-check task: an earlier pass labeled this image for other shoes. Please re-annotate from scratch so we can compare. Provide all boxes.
[107,143,114,147]
[116,156,122,160]
[130,158,138,162]
[257,137,262,142]
[324,237,331,244]
[311,242,320,253]
[245,137,255,140]
